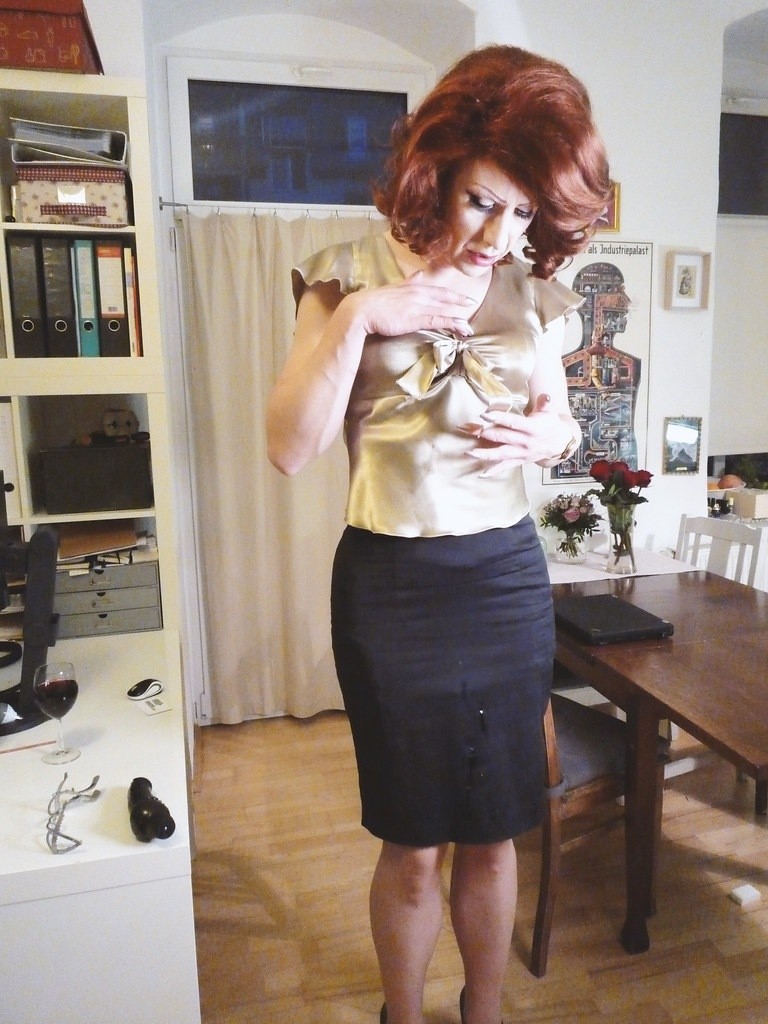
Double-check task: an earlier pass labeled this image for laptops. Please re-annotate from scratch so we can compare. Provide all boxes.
[551,591,675,644]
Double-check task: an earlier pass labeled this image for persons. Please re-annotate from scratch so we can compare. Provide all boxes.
[266,44,614,1024]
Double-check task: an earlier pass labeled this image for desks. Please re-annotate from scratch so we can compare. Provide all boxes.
[545,550,767,955]
[0,628,201,1024]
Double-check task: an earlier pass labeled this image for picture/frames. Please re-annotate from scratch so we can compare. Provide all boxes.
[665,250,711,310]
[662,416,702,474]
[586,183,621,232]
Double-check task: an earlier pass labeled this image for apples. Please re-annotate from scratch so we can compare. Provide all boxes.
[707,474,743,490]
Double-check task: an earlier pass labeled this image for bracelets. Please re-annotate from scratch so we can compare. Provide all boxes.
[560,436,576,460]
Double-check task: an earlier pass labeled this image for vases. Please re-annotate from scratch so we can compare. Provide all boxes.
[556,532,586,564]
[606,503,637,574]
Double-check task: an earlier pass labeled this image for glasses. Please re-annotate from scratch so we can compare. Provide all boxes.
[46,772,101,855]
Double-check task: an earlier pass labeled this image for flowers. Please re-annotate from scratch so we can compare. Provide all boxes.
[540,494,606,560]
[585,460,654,567]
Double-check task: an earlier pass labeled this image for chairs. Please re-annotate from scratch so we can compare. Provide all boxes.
[531,692,672,978]
[611,514,768,806]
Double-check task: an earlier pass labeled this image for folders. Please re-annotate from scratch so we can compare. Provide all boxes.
[94,240,130,357]
[10,145,113,166]
[8,237,45,358]
[41,237,78,357]
[74,238,101,356]
[7,117,127,164]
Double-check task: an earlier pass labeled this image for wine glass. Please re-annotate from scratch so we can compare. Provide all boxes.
[32,660,81,766]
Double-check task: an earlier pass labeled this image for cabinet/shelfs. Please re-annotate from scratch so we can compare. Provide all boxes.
[1,70,210,728]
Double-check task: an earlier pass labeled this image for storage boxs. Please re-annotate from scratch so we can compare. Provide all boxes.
[0,0,105,76]
[36,442,153,515]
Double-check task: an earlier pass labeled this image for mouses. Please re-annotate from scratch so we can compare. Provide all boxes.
[127,679,163,700]
